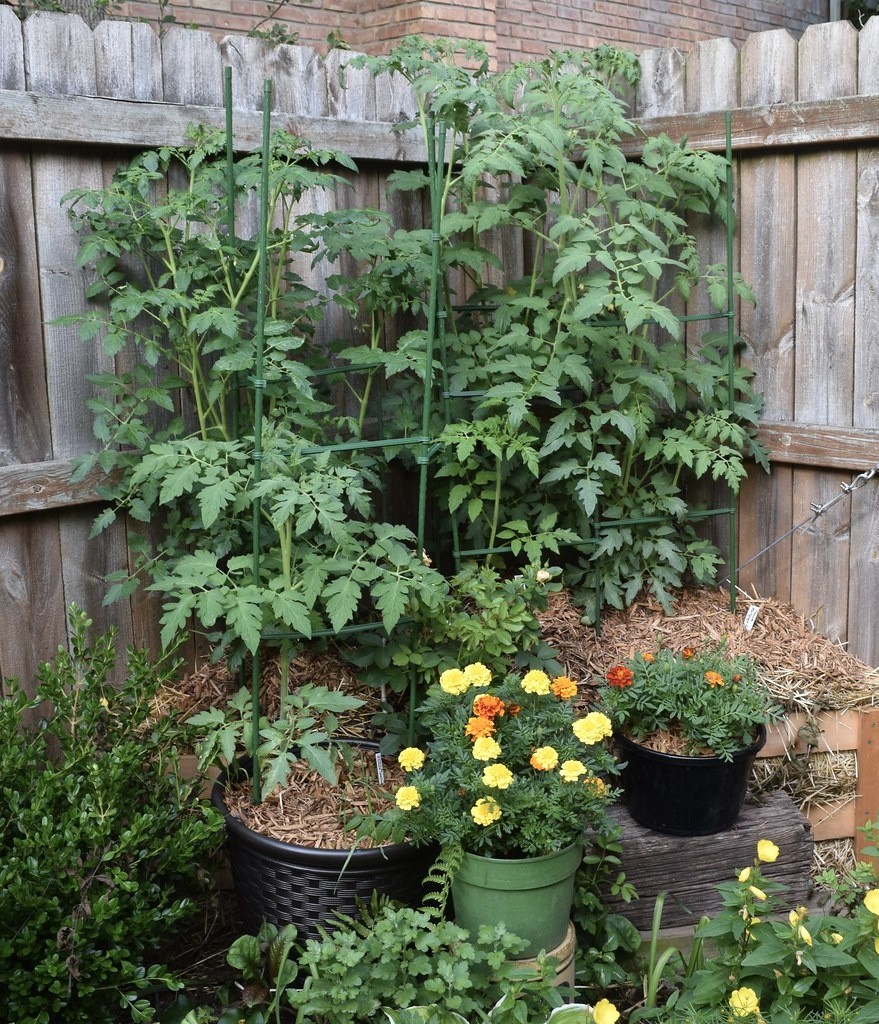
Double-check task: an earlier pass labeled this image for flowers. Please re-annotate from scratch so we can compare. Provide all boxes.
[596,632,785,764]
[346,662,630,859]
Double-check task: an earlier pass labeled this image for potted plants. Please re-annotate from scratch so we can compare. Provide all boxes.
[126,22,492,984]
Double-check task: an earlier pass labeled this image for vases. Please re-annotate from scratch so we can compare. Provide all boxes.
[614,707,766,837]
[450,837,585,959]
